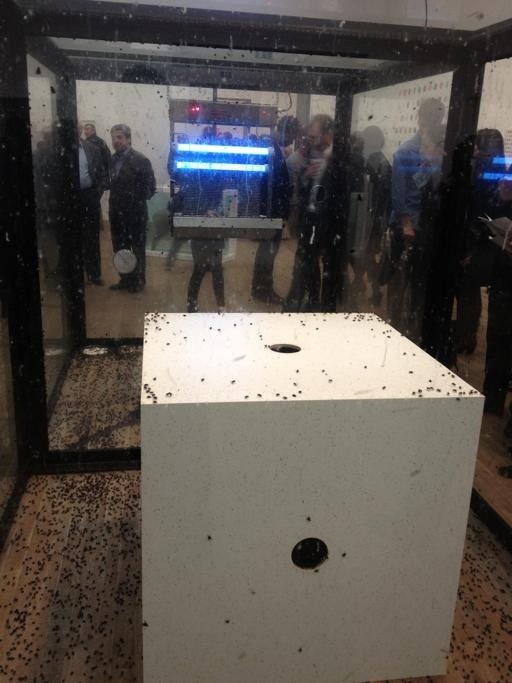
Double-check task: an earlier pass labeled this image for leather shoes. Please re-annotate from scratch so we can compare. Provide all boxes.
[106,275,148,294]
[251,286,285,304]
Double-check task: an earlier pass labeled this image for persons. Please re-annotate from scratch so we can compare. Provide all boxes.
[166,114,394,312]
[391,98,511,353]
[31,125,156,292]
